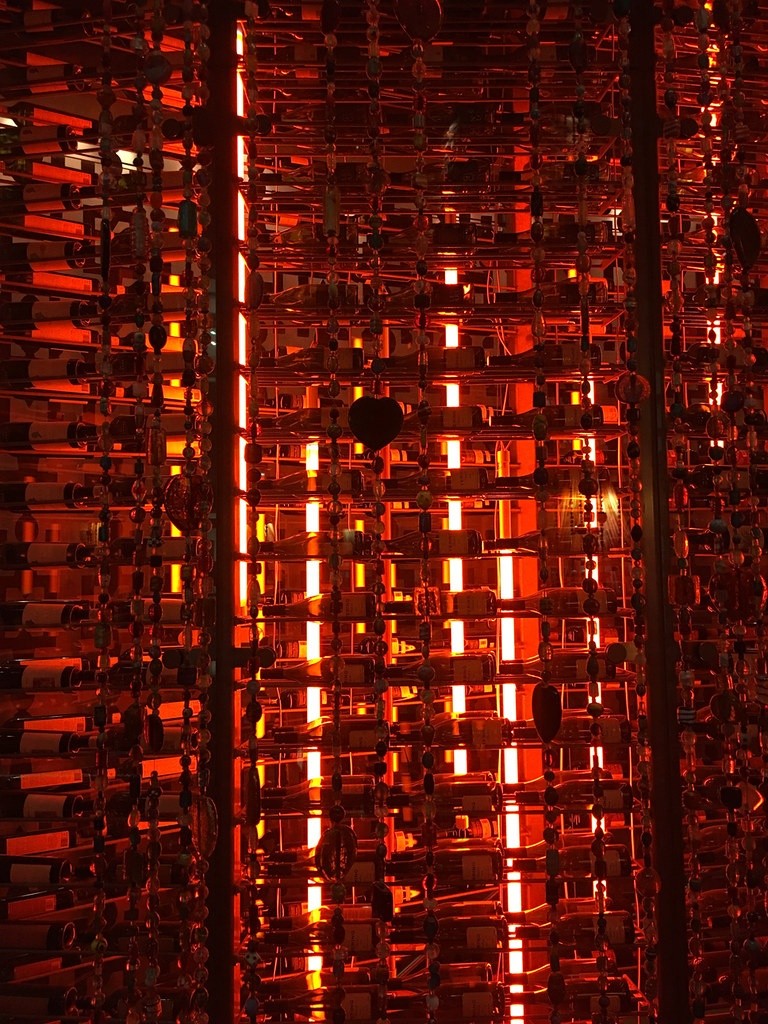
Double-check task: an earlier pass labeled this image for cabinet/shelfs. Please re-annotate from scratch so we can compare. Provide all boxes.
[0,0,768,1023]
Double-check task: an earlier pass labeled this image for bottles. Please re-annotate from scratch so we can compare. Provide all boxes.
[0,0,768,1024]
